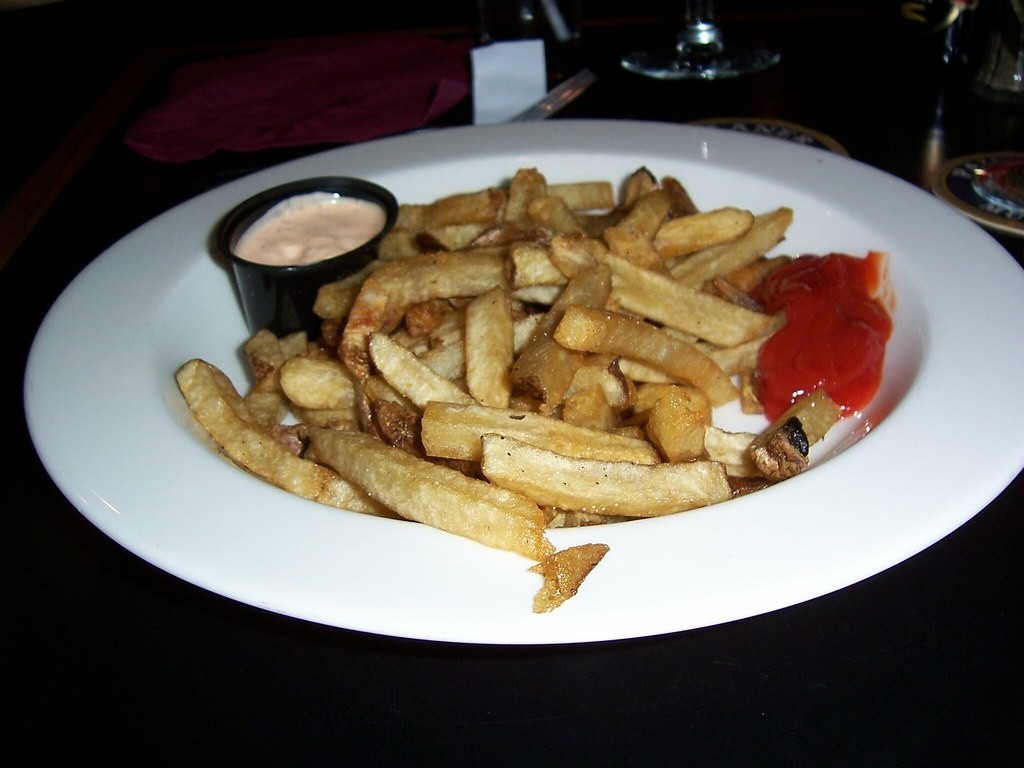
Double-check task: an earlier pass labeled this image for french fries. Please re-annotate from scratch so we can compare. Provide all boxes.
[175,167,844,559]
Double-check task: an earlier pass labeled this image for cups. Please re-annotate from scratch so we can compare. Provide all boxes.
[919,0,1024,238]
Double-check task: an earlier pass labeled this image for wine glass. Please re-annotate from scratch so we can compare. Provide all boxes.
[615,0,786,82]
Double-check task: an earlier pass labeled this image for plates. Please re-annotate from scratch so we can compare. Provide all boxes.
[22,115,1024,649]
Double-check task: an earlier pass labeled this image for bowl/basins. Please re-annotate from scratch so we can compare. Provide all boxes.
[216,176,399,341]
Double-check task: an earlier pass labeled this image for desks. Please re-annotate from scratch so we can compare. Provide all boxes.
[0,8,1024,768]
